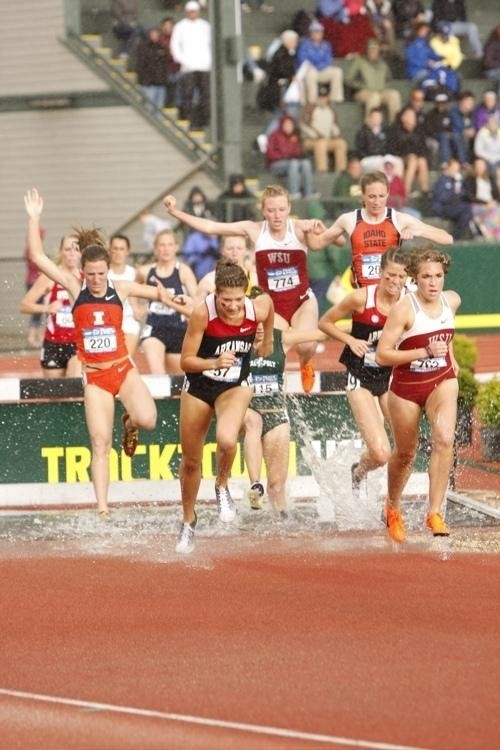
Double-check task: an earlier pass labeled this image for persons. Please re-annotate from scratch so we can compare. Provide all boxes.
[21,174,462,556]
[111,0,500,241]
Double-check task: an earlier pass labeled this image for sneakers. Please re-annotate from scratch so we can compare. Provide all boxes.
[300,361,315,393]
[426,512,450,536]
[215,482,236,523]
[350,462,362,497]
[382,496,406,543]
[248,481,264,511]
[121,414,137,457]
[175,511,198,556]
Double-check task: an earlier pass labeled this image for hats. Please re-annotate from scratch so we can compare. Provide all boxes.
[309,23,324,32]
[185,1,201,12]
[438,20,453,35]
